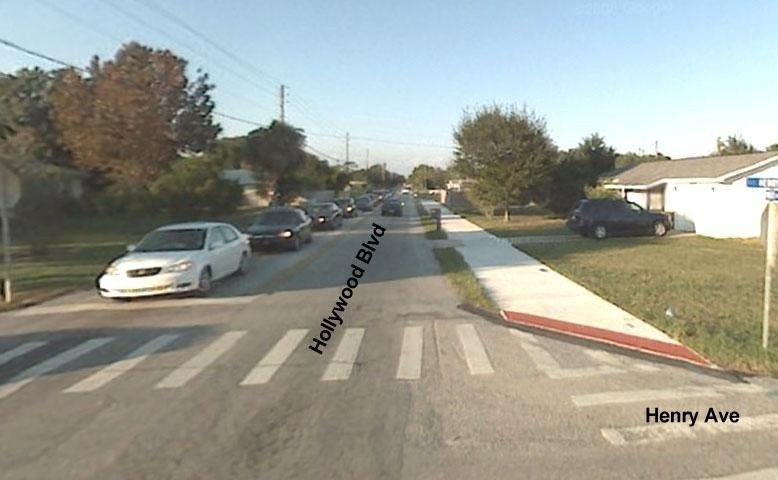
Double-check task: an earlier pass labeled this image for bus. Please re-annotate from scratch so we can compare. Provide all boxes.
[401,184,411,196]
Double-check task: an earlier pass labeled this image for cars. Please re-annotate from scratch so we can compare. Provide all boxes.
[97,221,253,303]
[381,199,403,218]
[244,192,393,253]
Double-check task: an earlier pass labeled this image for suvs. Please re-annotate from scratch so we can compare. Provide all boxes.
[566,198,672,240]
